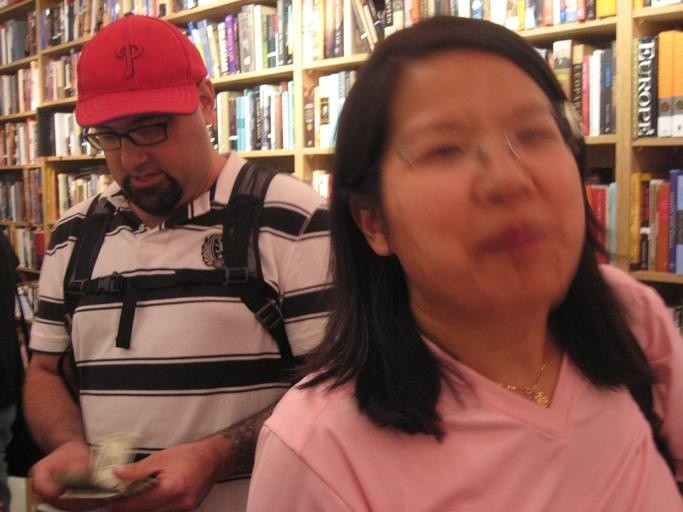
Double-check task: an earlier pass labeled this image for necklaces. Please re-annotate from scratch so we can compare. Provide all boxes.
[501,360,553,409]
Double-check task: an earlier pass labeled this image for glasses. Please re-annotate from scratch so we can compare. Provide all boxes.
[396,107,569,173]
[84,115,172,151]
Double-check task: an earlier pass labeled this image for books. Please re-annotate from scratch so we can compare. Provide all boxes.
[0,0,682,274]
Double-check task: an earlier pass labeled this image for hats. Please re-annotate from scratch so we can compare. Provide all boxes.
[74,12,209,129]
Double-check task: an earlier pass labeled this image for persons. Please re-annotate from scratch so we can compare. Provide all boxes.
[17,15,332,511]
[0,230,26,512]
[246,14,682,511]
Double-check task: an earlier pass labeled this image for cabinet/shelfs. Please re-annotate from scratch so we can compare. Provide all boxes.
[0,0,683,284]
